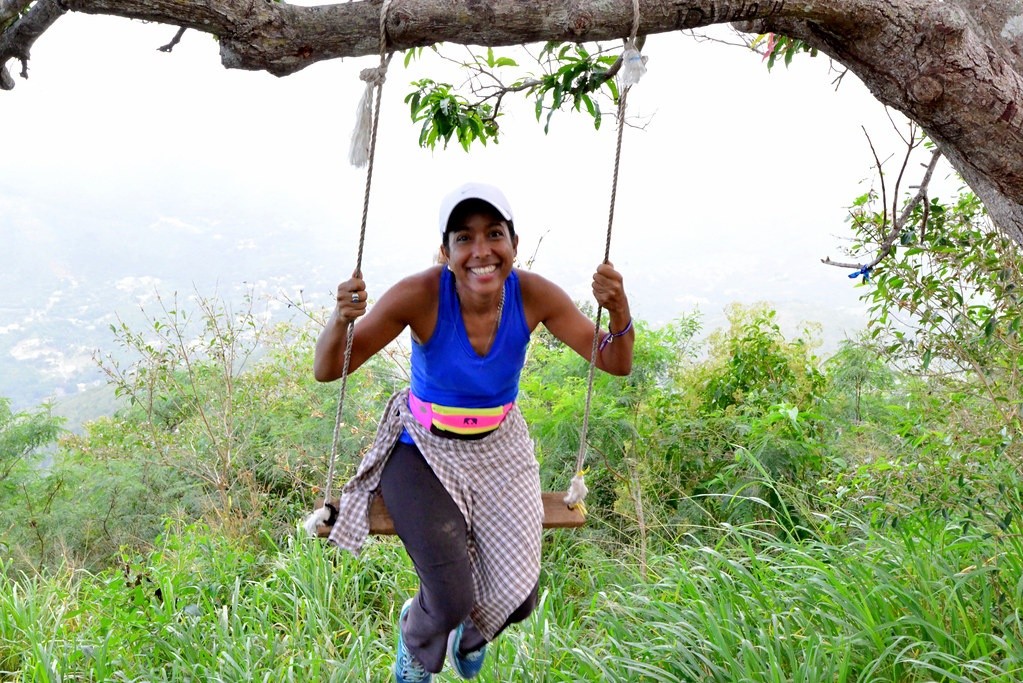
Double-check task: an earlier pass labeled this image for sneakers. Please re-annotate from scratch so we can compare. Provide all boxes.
[394,597,433,683]
[446,622,488,680]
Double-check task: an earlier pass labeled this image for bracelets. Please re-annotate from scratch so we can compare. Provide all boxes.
[596,316,633,351]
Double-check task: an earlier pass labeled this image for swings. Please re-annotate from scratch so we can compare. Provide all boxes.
[318,0,647,535]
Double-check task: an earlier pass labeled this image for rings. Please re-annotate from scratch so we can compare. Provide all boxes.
[350,290,359,303]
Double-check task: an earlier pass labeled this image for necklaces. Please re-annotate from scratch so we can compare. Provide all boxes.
[451,278,506,363]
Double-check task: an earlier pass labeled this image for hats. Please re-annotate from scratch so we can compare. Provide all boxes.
[438,182,515,245]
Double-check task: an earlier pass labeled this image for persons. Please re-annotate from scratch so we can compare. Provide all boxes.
[310,181,642,683]
